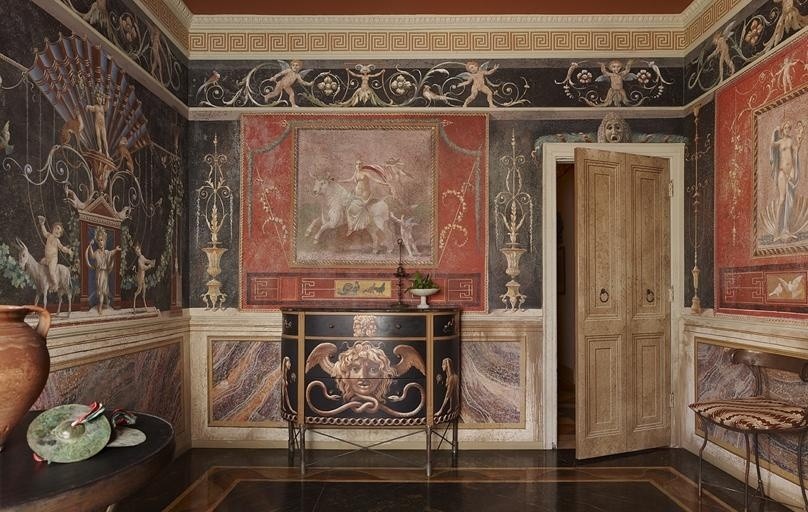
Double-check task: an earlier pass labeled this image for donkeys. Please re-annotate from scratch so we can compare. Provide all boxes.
[16,237,73,319]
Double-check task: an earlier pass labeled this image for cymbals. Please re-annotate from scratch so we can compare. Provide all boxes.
[27,402,147,464]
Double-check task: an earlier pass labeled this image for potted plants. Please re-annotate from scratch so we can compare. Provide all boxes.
[409,270,440,308]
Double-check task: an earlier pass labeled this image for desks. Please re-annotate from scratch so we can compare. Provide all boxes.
[0,409,174,511]
[280,305,461,477]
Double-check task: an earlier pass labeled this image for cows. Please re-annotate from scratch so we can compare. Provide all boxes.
[304,171,419,255]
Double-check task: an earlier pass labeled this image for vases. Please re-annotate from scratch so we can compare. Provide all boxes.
[1,303,51,451]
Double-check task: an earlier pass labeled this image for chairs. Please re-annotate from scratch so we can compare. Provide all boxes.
[687,349,808,512]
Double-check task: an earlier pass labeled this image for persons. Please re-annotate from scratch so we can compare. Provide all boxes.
[457,63,499,108]
[280,356,295,414]
[597,60,631,107]
[341,63,385,106]
[795,121,804,142]
[598,112,631,143]
[330,339,397,405]
[706,32,735,85]
[38,91,157,314]
[265,60,315,108]
[771,121,804,242]
[775,58,800,94]
[337,155,424,257]
[150,30,166,86]
[435,355,456,416]
